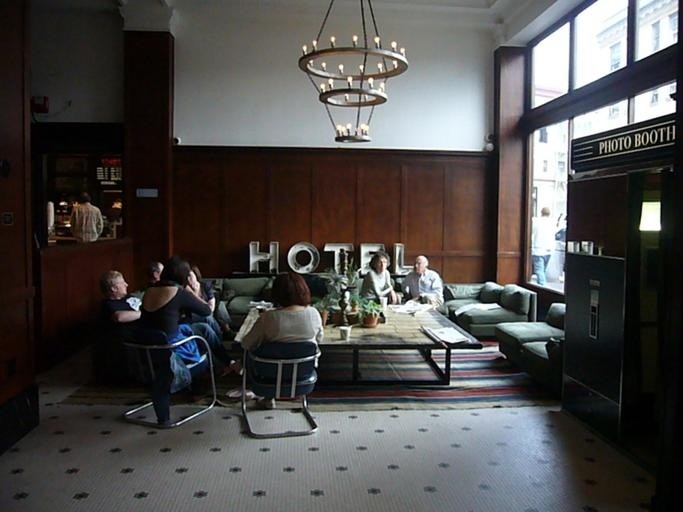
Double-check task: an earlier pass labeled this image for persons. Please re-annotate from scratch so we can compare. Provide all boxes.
[68,191,104,243]
[400,255,444,311]
[99,268,242,377]
[360,250,402,306]
[532,207,556,286]
[232,271,324,409]
[184,263,239,339]
[149,260,165,282]
[138,258,212,404]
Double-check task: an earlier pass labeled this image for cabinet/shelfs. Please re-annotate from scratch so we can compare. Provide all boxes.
[40,154,123,244]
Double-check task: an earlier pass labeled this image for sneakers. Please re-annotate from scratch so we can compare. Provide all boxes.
[256,396,276,409]
[231,357,244,375]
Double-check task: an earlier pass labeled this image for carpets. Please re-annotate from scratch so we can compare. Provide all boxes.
[60,336,558,411]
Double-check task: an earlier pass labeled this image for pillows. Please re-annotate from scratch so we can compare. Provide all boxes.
[480,281,530,315]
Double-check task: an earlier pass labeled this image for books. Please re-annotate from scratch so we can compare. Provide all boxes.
[422,325,474,345]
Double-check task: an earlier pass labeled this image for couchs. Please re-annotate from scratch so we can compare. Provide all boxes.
[495,302,565,382]
[198,271,402,336]
[443,281,538,341]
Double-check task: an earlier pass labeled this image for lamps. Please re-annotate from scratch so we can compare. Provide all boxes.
[297,0,409,143]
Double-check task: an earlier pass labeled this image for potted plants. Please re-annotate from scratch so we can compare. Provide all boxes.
[360,300,382,327]
[345,258,362,297]
[319,270,347,298]
[311,294,332,327]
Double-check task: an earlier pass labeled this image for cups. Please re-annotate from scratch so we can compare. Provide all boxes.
[339,326,350,340]
[380,297,387,308]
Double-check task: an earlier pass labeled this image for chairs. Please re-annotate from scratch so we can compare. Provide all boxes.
[240,342,318,438]
[119,323,217,429]
